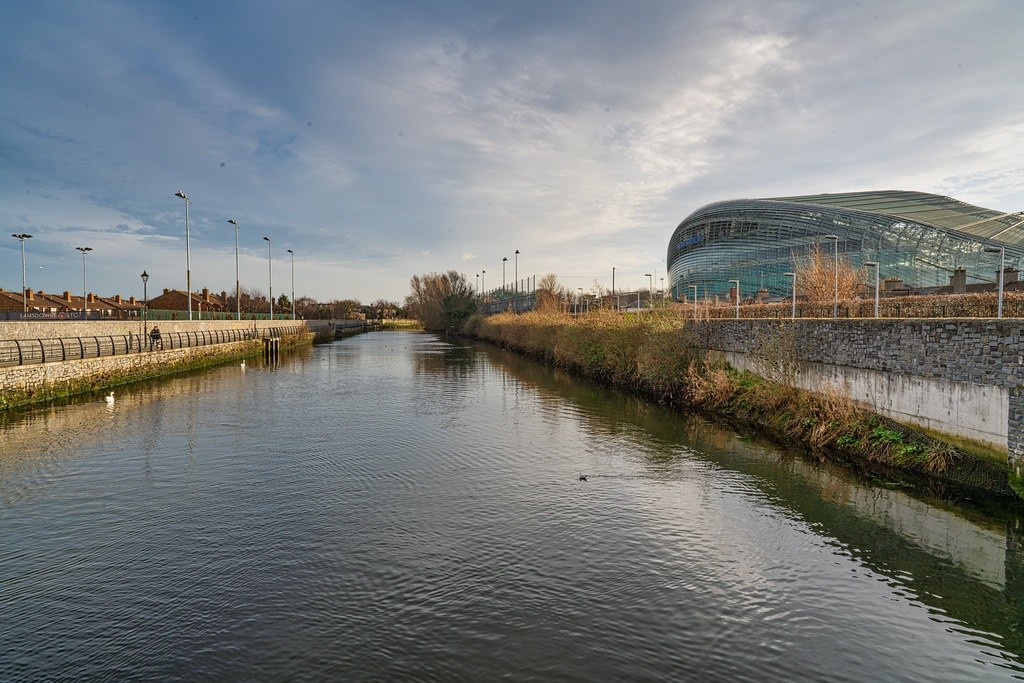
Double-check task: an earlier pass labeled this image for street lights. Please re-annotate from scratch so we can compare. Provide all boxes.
[12,233,33,315]
[174,190,193,320]
[784,272,796,319]
[76,246,92,316]
[141,270,150,348]
[263,237,273,320]
[982,244,1003,318]
[577,287,586,319]
[612,267,615,296]
[645,273,653,303]
[824,233,839,319]
[660,277,664,302]
[502,257,508,290]
[687,285,699,319]
[227,219,241,321]
[475,273,480,295]
[514,249,520,295]
[862,260,880,319]
[728,279,739,319]
[287,249,296,322]
[482,269,486,297]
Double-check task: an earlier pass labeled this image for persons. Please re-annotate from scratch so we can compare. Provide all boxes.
[150,326,161,350]
